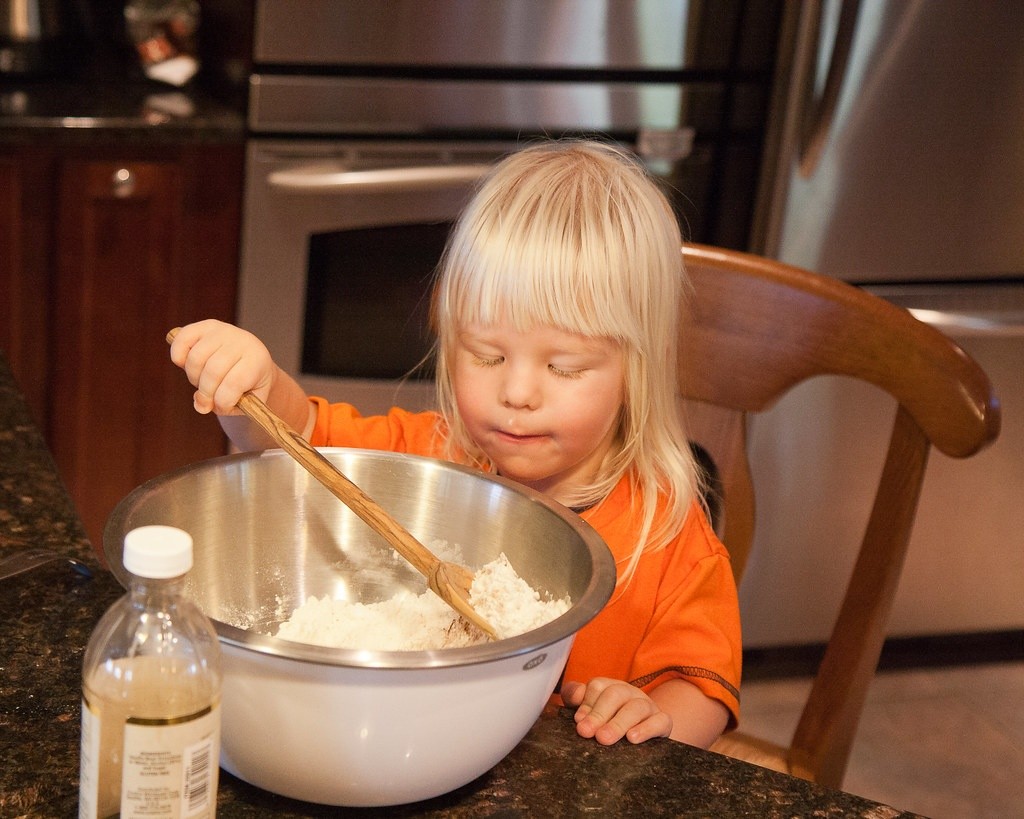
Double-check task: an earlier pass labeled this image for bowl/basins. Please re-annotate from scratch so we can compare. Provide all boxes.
[102,443,618,807]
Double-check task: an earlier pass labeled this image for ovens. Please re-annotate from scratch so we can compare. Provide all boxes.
[228,0,700,458]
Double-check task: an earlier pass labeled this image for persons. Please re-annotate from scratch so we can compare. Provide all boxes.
[166,138,741,748]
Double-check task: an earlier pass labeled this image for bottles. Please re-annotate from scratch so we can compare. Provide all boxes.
[78,525,223,819]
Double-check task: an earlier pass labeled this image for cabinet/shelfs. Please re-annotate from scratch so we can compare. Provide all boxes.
[0,98,247,572]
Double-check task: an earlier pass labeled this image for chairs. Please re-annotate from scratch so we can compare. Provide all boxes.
[673,242,1003,787]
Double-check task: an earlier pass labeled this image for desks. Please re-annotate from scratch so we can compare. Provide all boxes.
[0,372,929,819]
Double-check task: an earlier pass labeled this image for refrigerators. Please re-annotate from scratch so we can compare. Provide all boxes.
[692,0,1024,653]
